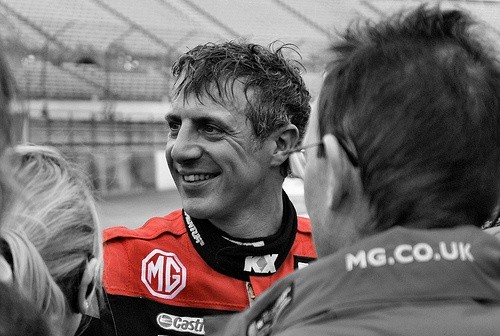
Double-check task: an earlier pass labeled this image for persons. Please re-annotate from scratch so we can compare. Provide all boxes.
[0,58,110,336]
[59,40,321,336]
[217,2,499,336]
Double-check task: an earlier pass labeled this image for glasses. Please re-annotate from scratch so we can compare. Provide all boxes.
[283,138,359,180]
